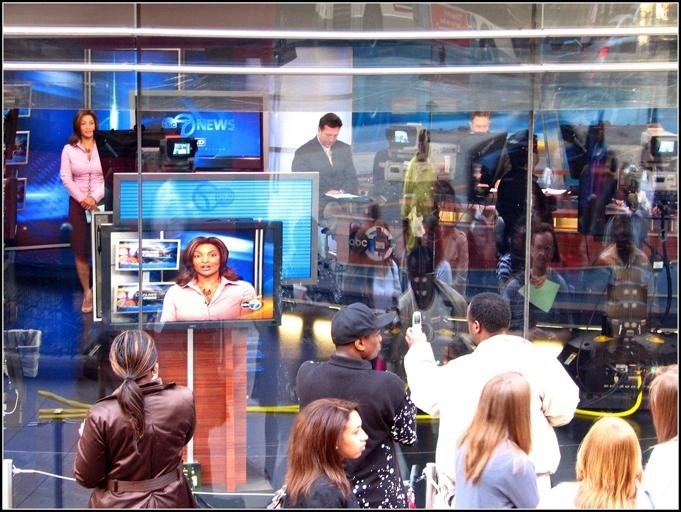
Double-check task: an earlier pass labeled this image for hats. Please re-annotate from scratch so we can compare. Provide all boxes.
[330,301,397,346]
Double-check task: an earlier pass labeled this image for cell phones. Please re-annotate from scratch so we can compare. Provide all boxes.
[412,311,421,332]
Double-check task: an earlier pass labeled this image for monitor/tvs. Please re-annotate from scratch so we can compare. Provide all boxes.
[99,218,282,329]
[129,90,269,168]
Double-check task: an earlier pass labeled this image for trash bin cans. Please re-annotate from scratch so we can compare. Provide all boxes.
[4,329,42,379]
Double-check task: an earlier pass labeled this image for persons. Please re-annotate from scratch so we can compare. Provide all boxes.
[73,330,197,508]
[291,113,359,222]
[264,293,678,509]
[159,129,659,487]
[60,109,105,314]
[468,111,492,134]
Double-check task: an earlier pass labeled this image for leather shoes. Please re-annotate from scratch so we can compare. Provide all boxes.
[80,289,94,315]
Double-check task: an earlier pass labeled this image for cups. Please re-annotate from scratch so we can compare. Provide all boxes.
[292,283,308,300]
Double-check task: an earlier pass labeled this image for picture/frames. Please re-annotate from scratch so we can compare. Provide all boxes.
[3,176,28,214]
[114,236,181,273]
[113,279,177,315]
[2,83,33,118]
[2,130,30,166]
